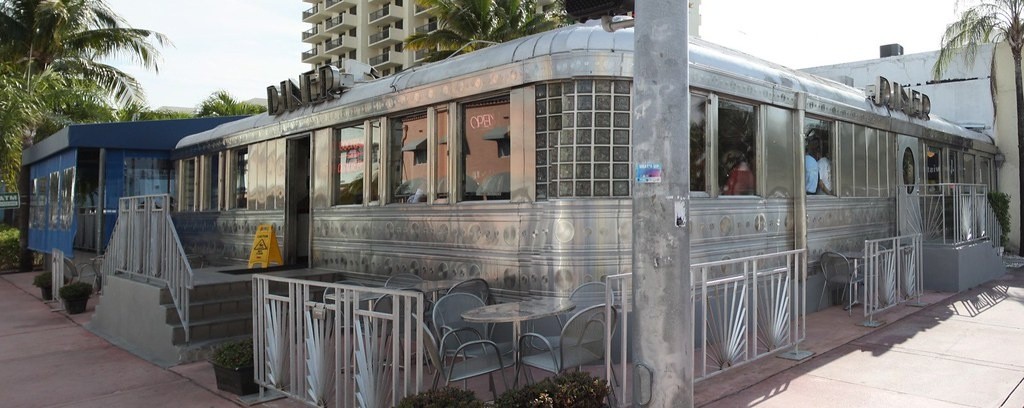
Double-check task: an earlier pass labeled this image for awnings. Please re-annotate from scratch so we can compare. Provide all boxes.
[401,127,511,152]
[394,173,510,197]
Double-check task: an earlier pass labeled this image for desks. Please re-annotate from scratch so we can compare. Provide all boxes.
[839,252,878,311]
[325,280,483,380]
[459,298,576,405]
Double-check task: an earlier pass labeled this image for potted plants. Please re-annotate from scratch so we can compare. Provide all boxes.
[58,281,93,315]
[32,272,70,300]
[203,337,270,396]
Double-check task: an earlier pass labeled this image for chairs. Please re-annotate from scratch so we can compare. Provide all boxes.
[432,292,530,392]
[841,245,888,307]
[816,252,864,318]
[524,281,620,387]
[411,313,514,395]
[370,288,427,373]
[512,303,618,405]
[384,273,436,361]
[423,278,490,368]
[323,280,379,375]
[92,255,106,291]
[63,256,92,285]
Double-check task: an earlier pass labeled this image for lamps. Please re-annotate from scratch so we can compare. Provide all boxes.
[994,154,1005,169]
[864,84,877,98]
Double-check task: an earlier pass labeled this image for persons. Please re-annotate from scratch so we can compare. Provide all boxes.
[721,145,834,196]
